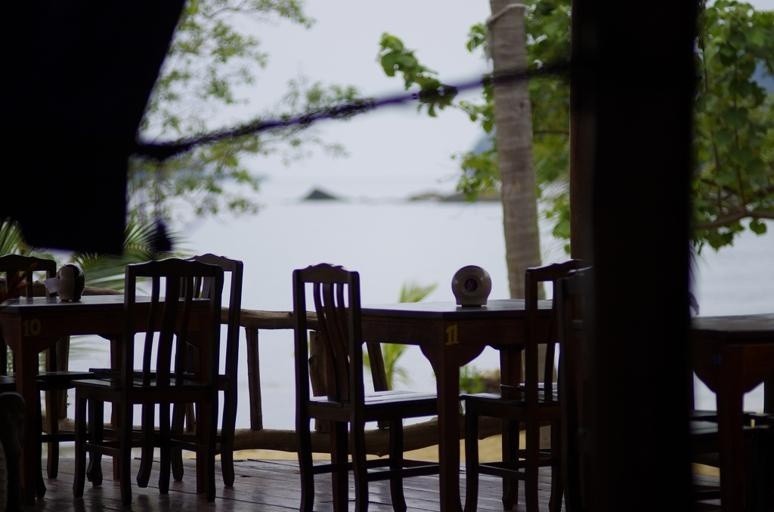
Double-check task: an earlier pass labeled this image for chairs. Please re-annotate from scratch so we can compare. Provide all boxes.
[0,252,774,512]
